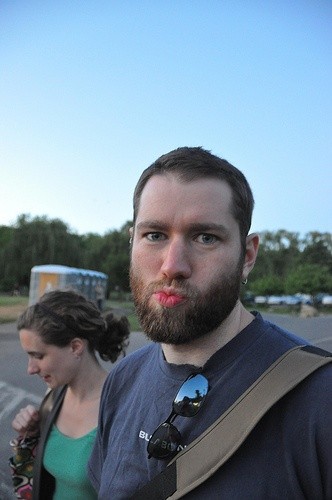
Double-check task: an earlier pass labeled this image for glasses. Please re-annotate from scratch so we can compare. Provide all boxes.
[147,371,210,460]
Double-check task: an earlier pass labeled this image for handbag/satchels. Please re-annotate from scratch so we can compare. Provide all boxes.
[9,436,40,500]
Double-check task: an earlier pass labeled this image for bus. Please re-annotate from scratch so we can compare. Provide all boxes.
[28,263,109,307]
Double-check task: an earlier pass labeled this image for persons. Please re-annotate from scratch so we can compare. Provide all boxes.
[95,280,104,312]
[87,147,332,500]
[0,288,129,500]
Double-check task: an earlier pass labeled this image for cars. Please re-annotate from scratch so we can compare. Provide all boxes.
[254,294,332,305]
